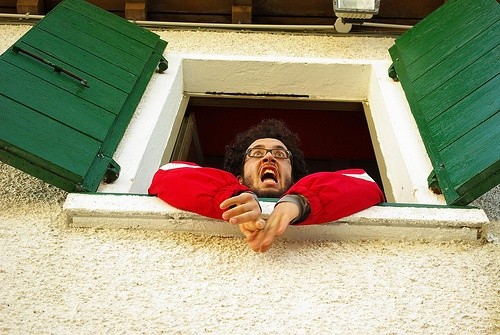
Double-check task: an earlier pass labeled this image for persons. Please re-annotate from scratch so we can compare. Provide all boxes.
[148,119,386,253]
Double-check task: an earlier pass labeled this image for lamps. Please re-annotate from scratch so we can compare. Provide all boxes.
[333,0,380,34]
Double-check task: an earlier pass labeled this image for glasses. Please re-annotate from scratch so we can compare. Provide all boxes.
[241,148,293,168]
